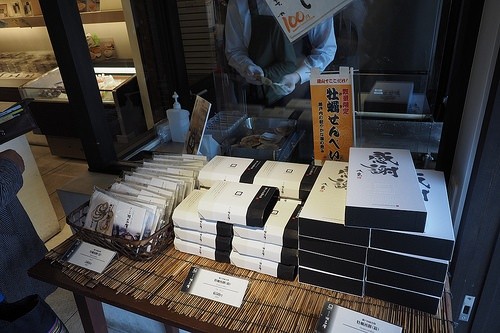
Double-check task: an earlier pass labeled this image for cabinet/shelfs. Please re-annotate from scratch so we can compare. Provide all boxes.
[0,0,190,129]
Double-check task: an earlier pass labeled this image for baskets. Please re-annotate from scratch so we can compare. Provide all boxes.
[65,197,175,261]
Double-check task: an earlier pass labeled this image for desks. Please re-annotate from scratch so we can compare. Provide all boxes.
[28,233,455,333]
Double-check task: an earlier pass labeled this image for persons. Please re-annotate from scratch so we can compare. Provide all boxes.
[224,0,337,109]
[0,148,59,305]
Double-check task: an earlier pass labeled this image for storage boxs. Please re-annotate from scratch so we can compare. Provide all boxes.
[364,81,413,113]
[345,148,428,232]
[298,160,455,315]
[172,155,324,282]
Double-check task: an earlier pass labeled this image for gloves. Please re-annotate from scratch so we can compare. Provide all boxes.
[227,53,264,85]
